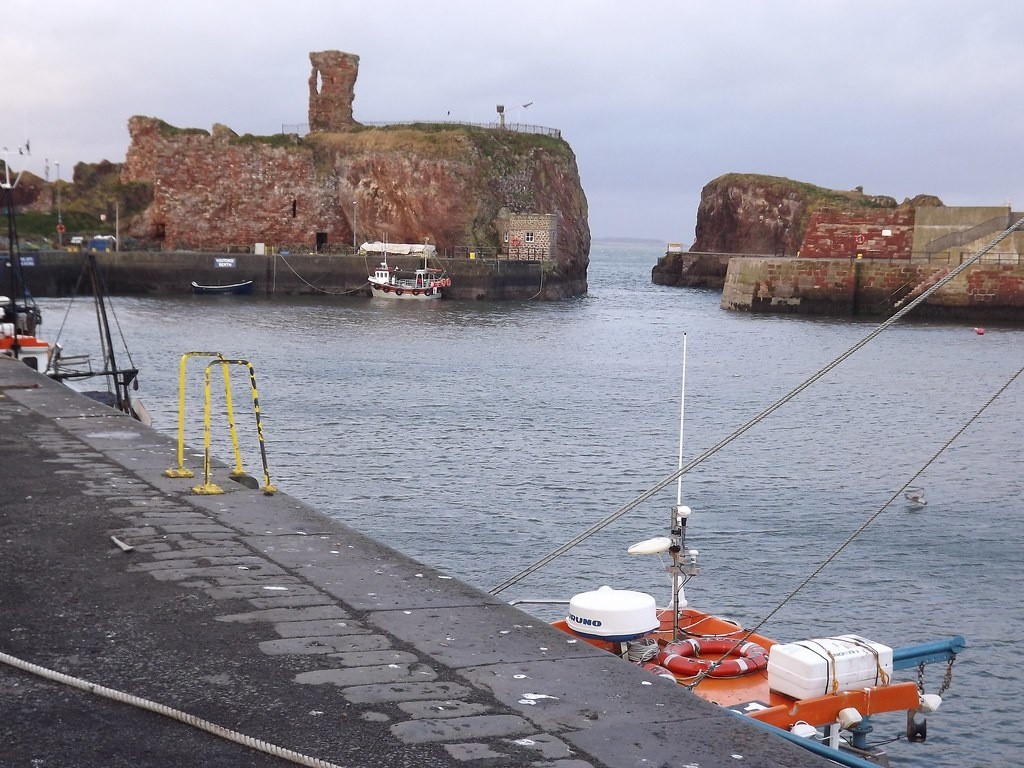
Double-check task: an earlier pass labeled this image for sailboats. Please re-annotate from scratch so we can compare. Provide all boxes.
[0,140,50,378]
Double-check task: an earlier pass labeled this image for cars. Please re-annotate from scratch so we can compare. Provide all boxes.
[20,241,40,251]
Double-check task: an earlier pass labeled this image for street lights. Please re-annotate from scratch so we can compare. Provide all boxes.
[54,161,62,245]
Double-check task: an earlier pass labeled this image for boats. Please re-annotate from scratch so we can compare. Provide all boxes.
[360,233,451,300]
[508,333,942,750]
[191,279,253,295]
[43,235,151,427]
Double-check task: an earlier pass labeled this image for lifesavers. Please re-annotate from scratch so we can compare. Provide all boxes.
[629,637,773,689]
[440,278,452,287]
[855,234,865,244]
[510,238,519,246]
[374,283,432,297]
[56,224,65,234]
